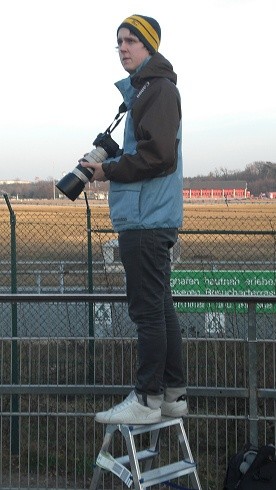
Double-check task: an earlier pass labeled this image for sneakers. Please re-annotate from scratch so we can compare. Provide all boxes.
[162,394,188,418]
[95,390,161,424]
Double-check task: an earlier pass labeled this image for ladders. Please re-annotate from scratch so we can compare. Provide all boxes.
[89,417,202,490]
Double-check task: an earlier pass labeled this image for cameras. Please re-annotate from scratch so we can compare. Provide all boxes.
[56,132,123,202]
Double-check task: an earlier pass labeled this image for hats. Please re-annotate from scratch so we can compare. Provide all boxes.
[117,14,161,56]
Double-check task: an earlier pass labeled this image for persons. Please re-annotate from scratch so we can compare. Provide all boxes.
[76,10,193,424]
